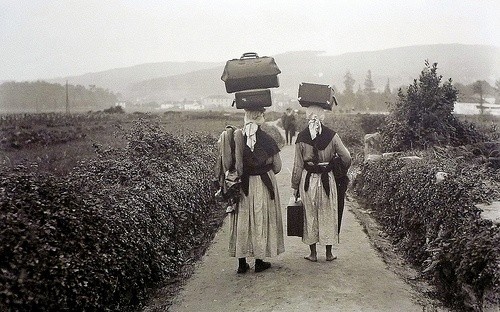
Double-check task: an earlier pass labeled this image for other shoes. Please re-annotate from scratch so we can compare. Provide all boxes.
[238,263,249,273]
[255,262,271,272]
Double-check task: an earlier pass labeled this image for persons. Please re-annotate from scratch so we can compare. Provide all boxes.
[281,109,295,145]
[214,107,285,274]
[290,104,351,262]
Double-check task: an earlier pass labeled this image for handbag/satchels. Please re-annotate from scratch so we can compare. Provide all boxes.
[221,52,281,93]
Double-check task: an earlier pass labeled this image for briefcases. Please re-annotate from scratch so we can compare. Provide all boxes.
[298,83,338,110]
[232,89,272,109]
[287,197,304,237]
